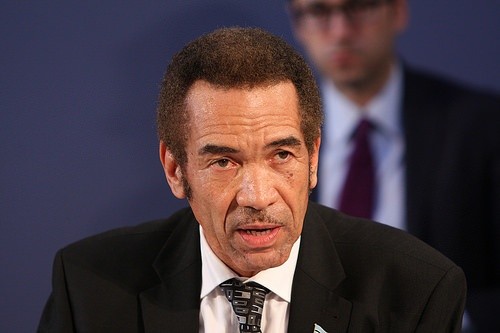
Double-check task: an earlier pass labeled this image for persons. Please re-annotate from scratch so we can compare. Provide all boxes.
[285,0,500,333]
[36,26,468,333]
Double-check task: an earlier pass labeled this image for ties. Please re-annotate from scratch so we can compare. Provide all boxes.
[337,118,380,220]
[218,277,271,333]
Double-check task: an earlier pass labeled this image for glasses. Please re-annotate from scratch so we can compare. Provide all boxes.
[292,1,394,29]
